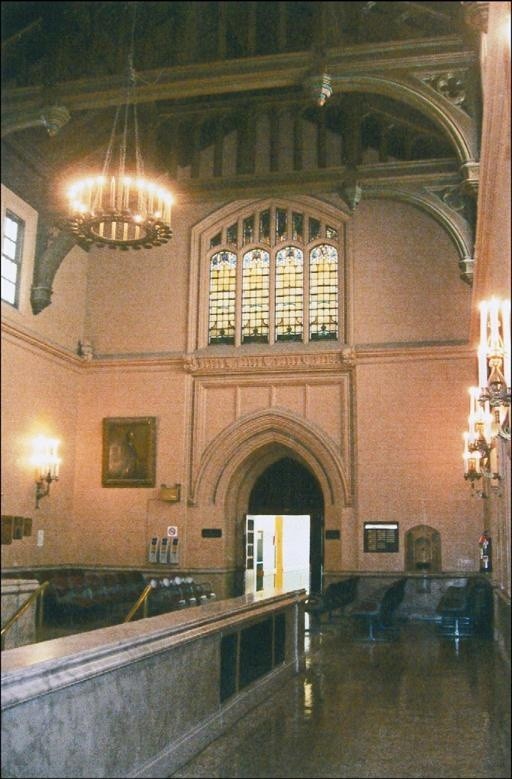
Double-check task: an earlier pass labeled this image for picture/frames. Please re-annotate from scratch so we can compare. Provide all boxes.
[101,416,156,489]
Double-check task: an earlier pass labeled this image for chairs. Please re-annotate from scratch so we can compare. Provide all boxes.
[0,569,215,626]
[300,576,489,643]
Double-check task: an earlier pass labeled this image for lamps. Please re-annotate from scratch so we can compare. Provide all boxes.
[28,434,61,509]
[64,0,177,250]
[463,297,511,499]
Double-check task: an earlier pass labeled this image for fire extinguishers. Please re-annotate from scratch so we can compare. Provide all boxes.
[478,529,492,572]
